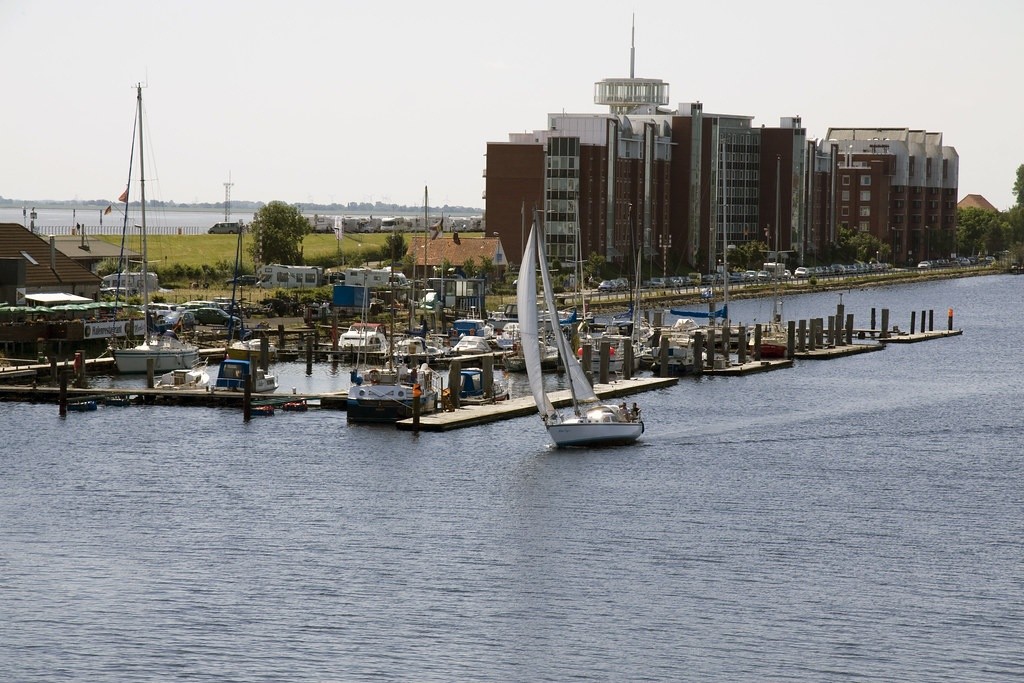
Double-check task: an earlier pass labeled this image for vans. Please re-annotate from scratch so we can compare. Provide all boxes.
[208,222,240,235]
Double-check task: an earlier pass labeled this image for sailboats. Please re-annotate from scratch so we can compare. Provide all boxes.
[107,65,200,376]
[516,203,645,445]
[748,156,789,359]
[347,268,440,423]
[340,185,707,377]
[690,139,751,349]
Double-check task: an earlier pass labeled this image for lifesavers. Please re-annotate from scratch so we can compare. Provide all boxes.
[369,370,382,383]
[451,329,458,336]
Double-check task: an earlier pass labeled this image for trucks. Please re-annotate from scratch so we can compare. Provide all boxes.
[344,268,407,287]
[763,262,785,281]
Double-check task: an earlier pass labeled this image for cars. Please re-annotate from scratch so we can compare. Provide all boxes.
[650,270,772,290]
[223,274,259,287]
[794,262,894,279]
[140,295,241,327]
[324,271,345,280]
[917,256,997,270]
[782,270,792,280]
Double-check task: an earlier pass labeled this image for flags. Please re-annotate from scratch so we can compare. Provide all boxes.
[104,205,111,215]
[118,188,130,204]
[702,287,712,298]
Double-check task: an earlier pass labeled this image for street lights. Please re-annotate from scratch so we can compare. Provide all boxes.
[892,226,897,267]
[134,223,142,318]
[877,251,879,262]
[925,225,931,262]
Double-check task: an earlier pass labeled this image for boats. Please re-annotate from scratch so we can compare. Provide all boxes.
[155,355,210,390]
[225,341,277,364]
[211,358,280,392]
[459,367,509,407]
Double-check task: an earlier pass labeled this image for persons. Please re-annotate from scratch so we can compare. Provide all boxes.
[75,223,81,235]
[617,402,639,423]
